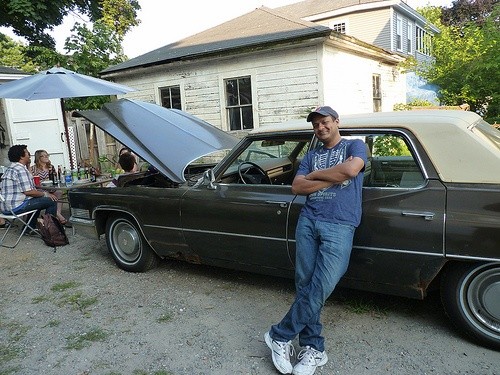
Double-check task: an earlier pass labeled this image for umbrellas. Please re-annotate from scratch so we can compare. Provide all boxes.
[0,66,141,170]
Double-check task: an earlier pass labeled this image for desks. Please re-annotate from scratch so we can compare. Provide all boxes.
[34,174,116,235]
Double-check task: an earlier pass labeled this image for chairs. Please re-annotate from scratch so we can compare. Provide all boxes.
[362,157,373,187]
[0,194,42,249]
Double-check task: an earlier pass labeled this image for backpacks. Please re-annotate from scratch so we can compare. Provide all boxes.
[39,214,70,247]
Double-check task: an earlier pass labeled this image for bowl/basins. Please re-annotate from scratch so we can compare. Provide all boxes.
[42,181,53,186]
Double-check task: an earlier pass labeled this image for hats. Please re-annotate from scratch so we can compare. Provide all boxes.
[307,106,338,122]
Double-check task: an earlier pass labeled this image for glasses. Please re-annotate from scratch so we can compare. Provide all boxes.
[40,155,49,158]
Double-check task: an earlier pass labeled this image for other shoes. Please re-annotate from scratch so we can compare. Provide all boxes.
[21,230,40,236]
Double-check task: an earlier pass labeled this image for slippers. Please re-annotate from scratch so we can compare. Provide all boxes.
[59,218,68,224]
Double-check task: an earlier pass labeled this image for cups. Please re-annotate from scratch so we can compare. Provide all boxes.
[33,176,40,186]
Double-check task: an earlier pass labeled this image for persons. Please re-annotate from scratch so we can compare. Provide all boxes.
[0,145,68,236]
[264,105,369,375]
[105,148,139,187]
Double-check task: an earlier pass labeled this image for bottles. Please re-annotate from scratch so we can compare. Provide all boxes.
[49,164,96,186]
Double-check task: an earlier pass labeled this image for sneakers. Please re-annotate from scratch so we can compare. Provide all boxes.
[292,346,328,375]
[264,331,296,375]
[0,221,18,229]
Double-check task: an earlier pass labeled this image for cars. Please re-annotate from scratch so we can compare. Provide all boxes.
[66,98,500,348]
